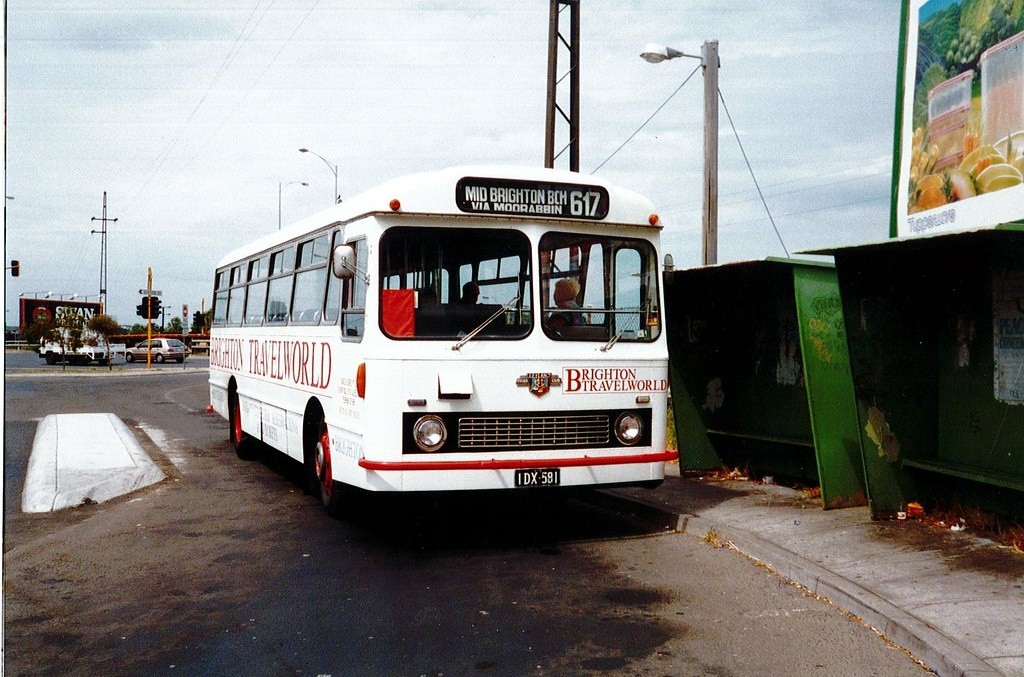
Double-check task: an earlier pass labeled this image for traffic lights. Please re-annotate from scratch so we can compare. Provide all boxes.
[136,297,148,320]
[149,295,162,319]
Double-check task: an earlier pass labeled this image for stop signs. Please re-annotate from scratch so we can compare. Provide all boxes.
[182,307,189,317]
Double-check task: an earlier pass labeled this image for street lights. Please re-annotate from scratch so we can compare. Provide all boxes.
[298,146,340,207]
[638,38,719,268]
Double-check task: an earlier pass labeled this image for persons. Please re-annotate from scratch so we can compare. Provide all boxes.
[546,278,586,327]
[460,282,479,305]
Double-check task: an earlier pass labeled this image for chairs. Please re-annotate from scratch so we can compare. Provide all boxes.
[243,308,339,324]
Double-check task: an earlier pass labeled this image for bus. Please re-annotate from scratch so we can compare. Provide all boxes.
[209,168,670,518]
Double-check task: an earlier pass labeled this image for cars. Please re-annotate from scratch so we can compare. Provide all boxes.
[39,324,116,367]
[122,337,193,364]
